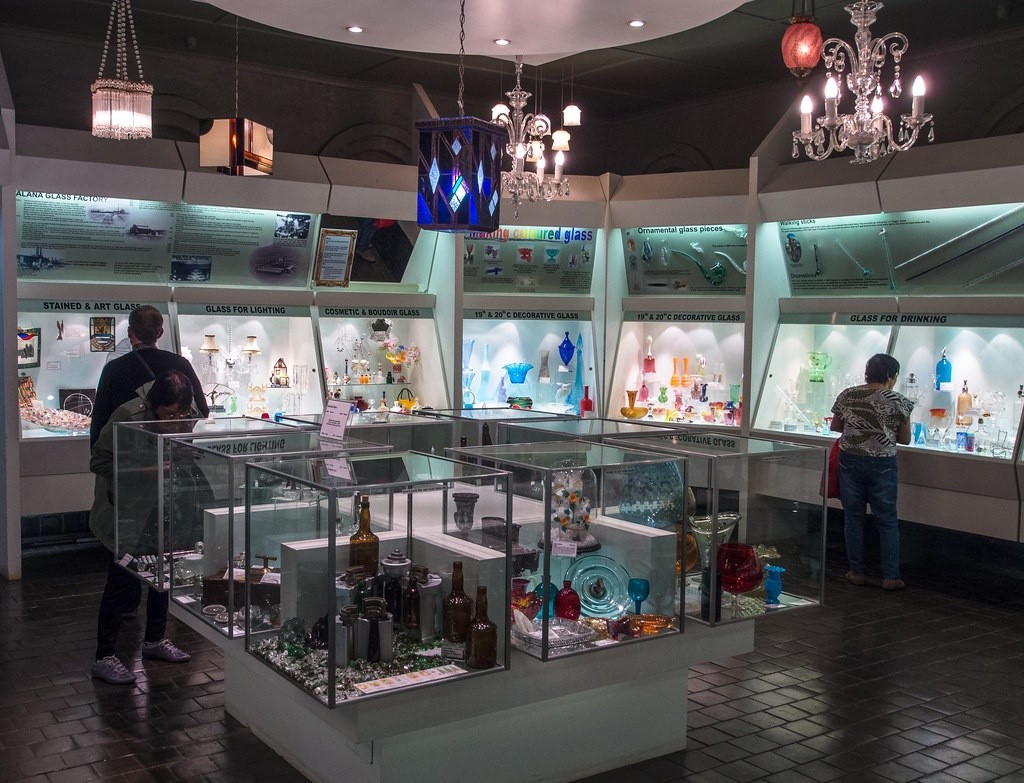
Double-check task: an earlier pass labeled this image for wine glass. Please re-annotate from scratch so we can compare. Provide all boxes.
[628,577,650,615]
[717,541,762,620]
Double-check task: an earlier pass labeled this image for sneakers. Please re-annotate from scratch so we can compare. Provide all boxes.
[142,640,191,663]
[92,656,135,684]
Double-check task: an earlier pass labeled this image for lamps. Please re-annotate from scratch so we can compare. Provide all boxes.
[198,333,262,376]
[781,0,935,164]
[491,52,581,219]
[414,0,508,234]
[91,0,154,140]
[199,16,274,177]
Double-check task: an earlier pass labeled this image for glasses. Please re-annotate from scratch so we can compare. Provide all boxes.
[157,404,191,420]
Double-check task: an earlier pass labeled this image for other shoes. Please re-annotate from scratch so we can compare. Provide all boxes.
[883,577,905,592]
[846,570,864,586]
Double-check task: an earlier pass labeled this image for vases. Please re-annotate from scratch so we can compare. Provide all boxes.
[765,565,786,605]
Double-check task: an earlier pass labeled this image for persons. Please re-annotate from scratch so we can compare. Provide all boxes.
[90,372,205,682]
[832,353,915,588]
[90,306,208,446]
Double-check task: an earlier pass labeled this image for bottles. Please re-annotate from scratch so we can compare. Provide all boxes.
[658,387,668,403]
[723,399,742,426]
[509,575,581,624]
[386,371,392,384]
[360,375,368,383]
[444,562,473,643]
[670,356,690,387]
[385,575,420,630]
[957,380,972,426]
[464,586,497,669]
[618,451,685,528]
[580,385,592,417]
[350,494,379,576]
[936,346,952,390]
